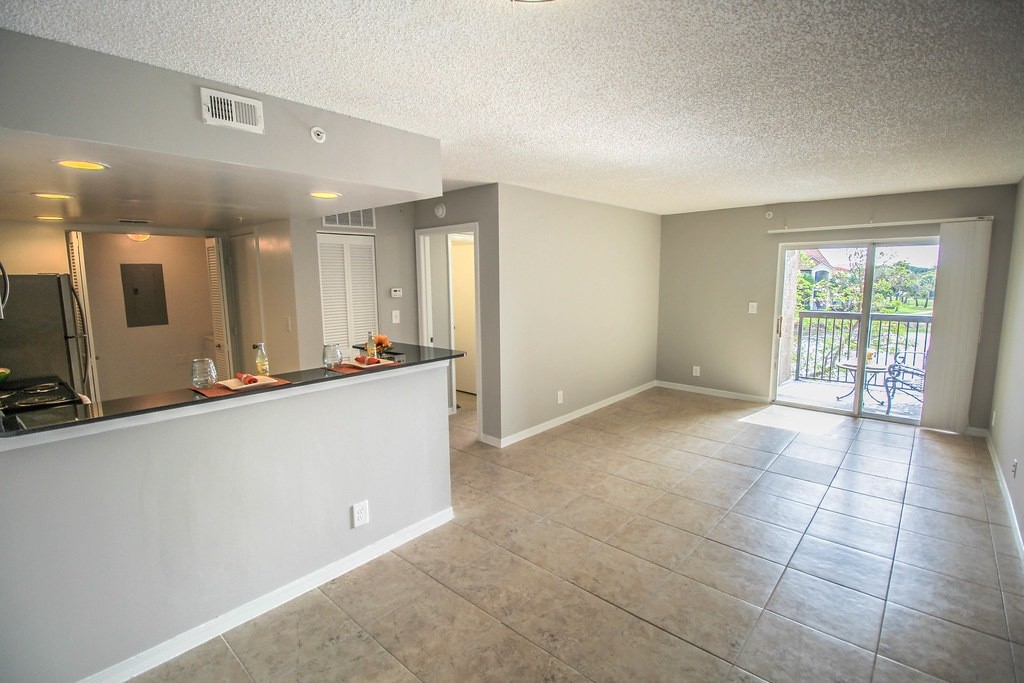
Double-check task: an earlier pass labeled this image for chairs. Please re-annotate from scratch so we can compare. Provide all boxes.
[884,351,927,415]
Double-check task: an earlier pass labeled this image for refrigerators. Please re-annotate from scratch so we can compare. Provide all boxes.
[0,272,89,403]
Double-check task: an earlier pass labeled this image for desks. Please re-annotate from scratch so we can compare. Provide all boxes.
[836,360,888,405]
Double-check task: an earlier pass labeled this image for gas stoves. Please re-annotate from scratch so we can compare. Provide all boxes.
[0,375,79,420]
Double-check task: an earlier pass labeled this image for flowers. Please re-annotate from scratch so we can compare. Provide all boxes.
[365,335,392,359]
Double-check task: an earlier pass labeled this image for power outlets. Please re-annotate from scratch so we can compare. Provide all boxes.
[353,501,368,528]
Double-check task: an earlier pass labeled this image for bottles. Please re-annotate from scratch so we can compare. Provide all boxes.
[255,342,271,376]
[367,330,377,359]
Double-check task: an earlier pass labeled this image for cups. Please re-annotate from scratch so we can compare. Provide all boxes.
[866,349,877,360]
[322,342,343,369]
[191,357,220,389]
